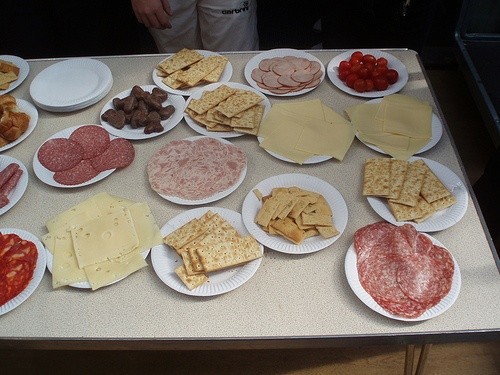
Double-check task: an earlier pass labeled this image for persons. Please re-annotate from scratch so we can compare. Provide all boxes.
[132,0,259,54]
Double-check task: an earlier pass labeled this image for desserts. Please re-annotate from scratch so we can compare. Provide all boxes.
[101,85,175,134]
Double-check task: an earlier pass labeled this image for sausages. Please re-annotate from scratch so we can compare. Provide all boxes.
[38,125,135,185]
[251,55,323,94]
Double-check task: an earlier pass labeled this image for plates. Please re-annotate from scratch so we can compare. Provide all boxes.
[32,125,119,189]
[244,48,326,98]
[152,49,233,98]
[327,49,408,98]
[29,58,113,114]
[0,155,28,215]
[0,99,38,152]
[0,227,47,315]
[100,85,185,140]
[241,173,349,255]
[367,156,469,232]
[0,54,30,95]
[257,137,332,165]
[356,98,443,155]
[184,82,271,138]
[156,135,247,206]
[44,247,151,289]
[150,207,264,298]
[344,233,462,322]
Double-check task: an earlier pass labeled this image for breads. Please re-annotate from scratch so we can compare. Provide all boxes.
[0,93,30,148]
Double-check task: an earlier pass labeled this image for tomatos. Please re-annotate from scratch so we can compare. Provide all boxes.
[339,52,399,92]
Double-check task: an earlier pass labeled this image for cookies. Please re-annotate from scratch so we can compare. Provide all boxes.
[163,209,264,291]
[184,84,265,136]
[252,186,339,245]
[0,59,19,90]
[156,48,228,91]
[362,156,458,223]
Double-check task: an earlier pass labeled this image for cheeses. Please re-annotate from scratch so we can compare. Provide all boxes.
[256,98,356,165]
[41,191,164,291]
[345,94,433,161]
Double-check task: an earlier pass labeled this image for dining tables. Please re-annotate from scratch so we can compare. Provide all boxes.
[0,48,500,375]
